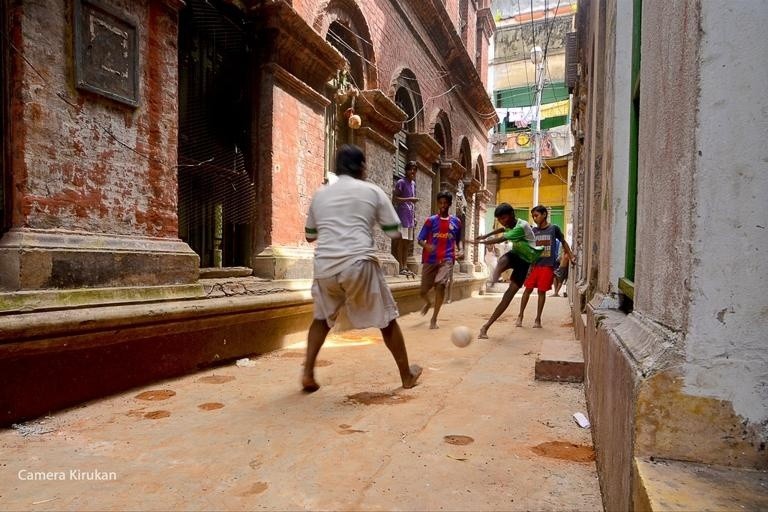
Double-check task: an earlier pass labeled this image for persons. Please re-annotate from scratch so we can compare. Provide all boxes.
[416,190,465,329]
[301,145,423,391]
[471,202,577,339]
[392,161,420,275]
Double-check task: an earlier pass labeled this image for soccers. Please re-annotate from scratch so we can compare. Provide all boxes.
[451,326,472,347]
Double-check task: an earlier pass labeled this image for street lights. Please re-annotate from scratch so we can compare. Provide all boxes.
[528,44,547,207]
